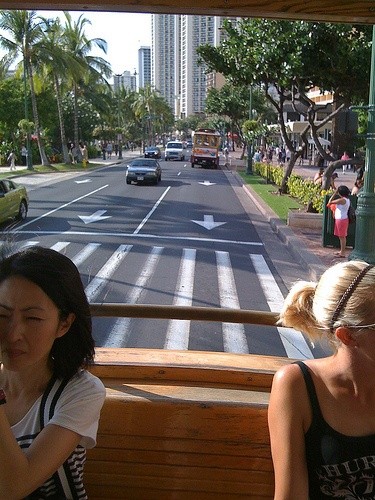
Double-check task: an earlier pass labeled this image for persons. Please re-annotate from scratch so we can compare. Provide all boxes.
[314,168,339,190]
[327,185,351,258]
[268,260,375,500]
[341,152,350,173]
[0,246,106,500]
[68,139,134,166]
[7,145,28,171]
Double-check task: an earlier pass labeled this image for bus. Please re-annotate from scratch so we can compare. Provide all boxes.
[191,128,221,169]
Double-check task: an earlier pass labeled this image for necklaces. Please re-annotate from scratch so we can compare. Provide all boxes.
[251,144,304,166]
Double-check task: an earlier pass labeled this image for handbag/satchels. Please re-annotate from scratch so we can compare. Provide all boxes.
[347,206,356,227]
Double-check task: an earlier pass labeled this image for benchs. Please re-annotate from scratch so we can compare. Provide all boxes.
[77,302,310,500]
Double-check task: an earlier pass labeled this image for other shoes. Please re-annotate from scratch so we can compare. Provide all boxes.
[334,251,345,257]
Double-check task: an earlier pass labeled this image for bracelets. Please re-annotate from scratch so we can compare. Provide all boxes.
[0,390,8,405]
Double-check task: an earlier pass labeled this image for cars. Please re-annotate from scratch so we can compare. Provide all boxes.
[126,158,161,186]
[145,146,161,158]
[0,178,29,224]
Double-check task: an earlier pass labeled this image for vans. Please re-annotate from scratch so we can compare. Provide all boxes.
[164,141,186,161]
